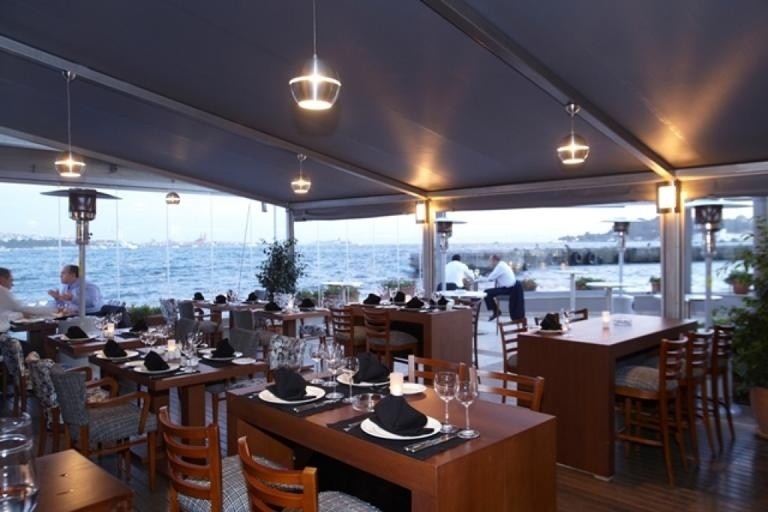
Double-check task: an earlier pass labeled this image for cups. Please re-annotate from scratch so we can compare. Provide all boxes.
[390,372,404,397]
[0,433,42,511]
[1,410,32,437]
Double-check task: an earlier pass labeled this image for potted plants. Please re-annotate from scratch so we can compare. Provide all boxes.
[256,236,308,310]
[724,271,755,295]
[711,213,767,445]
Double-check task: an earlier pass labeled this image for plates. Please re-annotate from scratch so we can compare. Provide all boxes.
[360,414,442,440]
[401,381,427,395]
[232,357,256,365]
[258,386,325,405]
[202,352,243,361]
[539,327,567,333]
[401,305,422,311]
[335,371,393,389]
[96,349,139,360]
[61,334,98,343]
[133,362,179,375]
[198,347,217,355]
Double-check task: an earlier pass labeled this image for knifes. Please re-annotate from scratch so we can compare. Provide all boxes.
[292,401,337,412]
[412,435,454,453]
[403,436,448,451]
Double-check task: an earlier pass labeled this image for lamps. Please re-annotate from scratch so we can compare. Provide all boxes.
[412,199,432,225]
[53,69,88,181]
[555,102,590,166]
[287,0,343,112]
[655,178,683,215]
[166,179,180,206]
[290,153,312,196]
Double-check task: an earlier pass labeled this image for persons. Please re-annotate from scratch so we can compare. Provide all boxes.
[1,266,69,361]
[483,254,517,321]
[437,254,475,294]
[45,265,104,317]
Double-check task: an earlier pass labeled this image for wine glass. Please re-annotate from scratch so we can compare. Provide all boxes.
[110,310,122,334]
[322,344,339,387]
[341,355,360,404]
[139,332,151,358]
[452,373,481,439]
[324,349,343,400]
[178,339,192,370]
[146,333,158,351]
[95,316,108,341]
[26,296,49,310]
[432,371,460,434]
[184,343,196,373]
[188,330,203,359]
[307,344,324,385]
